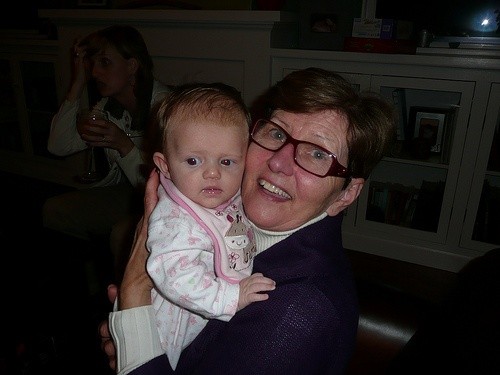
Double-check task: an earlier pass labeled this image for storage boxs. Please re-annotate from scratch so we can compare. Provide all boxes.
[344,37,416,54]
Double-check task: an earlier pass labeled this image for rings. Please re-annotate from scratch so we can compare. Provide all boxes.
[74,52,79,57]
[102,135,105,142]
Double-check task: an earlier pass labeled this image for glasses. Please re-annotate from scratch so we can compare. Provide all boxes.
[250,118,352,179]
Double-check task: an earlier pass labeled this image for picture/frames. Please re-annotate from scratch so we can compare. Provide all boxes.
[404,105,458,161]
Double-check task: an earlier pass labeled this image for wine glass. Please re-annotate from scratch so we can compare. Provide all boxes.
[77,108,108,181]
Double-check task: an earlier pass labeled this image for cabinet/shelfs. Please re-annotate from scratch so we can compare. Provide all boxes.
[271,48,500,273]
[0,10,299,189]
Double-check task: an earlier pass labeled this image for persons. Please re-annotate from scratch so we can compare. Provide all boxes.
[145,83,276,371]
[46,24,176,277]
[98,68,396,375]
